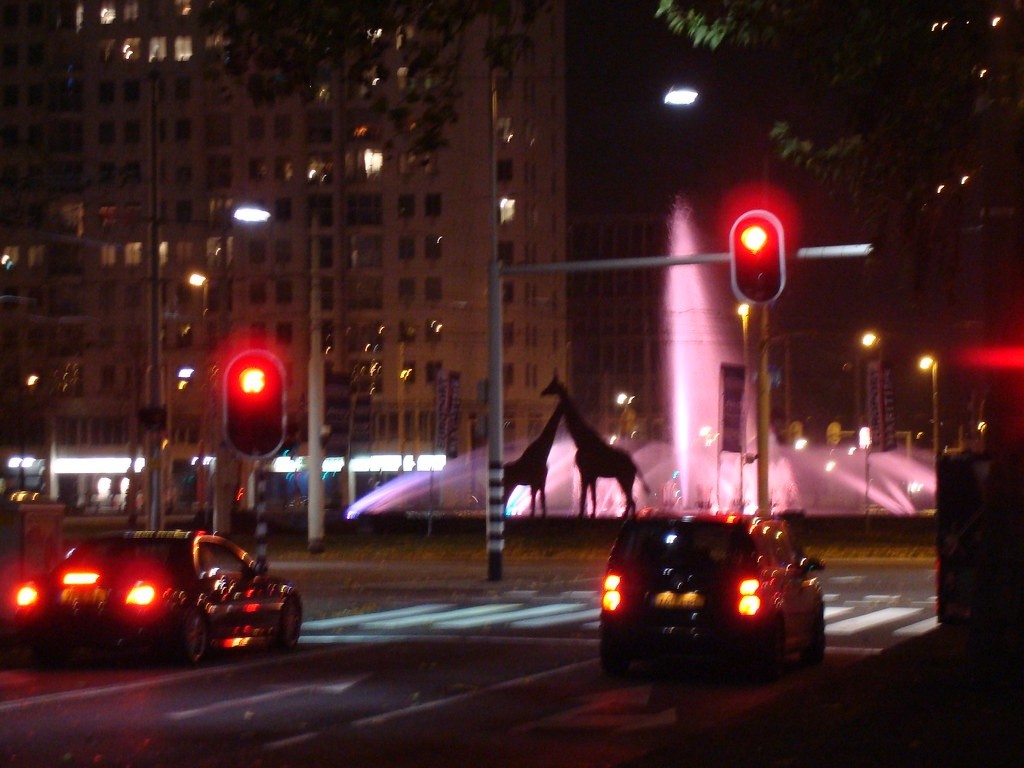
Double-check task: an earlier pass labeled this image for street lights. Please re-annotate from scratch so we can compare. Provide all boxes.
[919,356,940,461]
[735,302,750,372]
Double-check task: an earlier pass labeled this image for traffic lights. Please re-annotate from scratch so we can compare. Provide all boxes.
[730,210,786,307]
[222,348,286,460]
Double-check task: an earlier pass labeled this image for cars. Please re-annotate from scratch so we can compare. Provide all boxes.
[0,528,304,670]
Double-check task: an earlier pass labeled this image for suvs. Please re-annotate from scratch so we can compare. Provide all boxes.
[599,506,826,684]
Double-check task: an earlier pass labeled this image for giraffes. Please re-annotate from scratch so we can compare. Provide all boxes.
[540,367,650,518]
[503,390,572,516]
